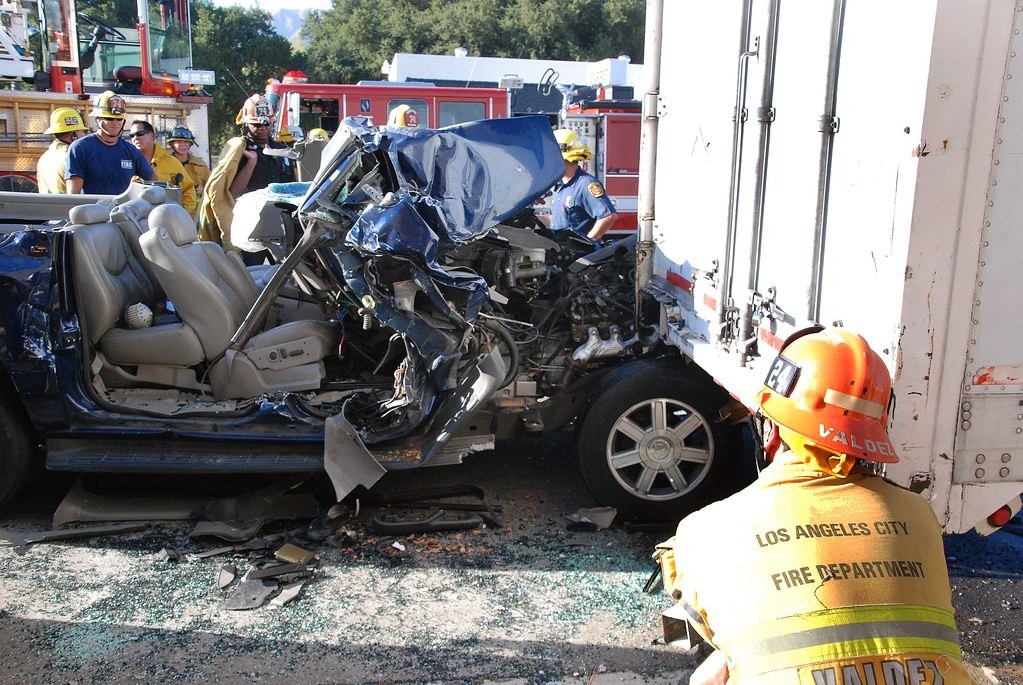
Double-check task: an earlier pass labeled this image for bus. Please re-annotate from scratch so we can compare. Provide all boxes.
[1,0,217,231]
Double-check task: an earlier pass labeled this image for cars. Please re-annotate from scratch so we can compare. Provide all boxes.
[0,112,763,537]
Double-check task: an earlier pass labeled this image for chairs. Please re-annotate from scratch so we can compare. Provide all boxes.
[113,24,178,94]
[139,204,336,401]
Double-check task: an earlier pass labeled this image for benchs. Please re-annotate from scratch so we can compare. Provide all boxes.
[63,186,212,391]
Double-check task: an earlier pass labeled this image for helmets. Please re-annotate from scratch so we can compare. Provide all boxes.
[552,129,592,162]
[755,326,900,463]
[88,91,127,119]
[166,126,194,146]
[236,94,276,124]
[43,107,88,134]
[387,105,420,129]
[308,129,330,143]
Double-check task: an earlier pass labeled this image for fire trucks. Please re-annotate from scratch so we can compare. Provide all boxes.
[259,70,641,248]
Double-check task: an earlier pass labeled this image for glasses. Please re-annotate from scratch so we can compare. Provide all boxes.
[251,123,270,127]
[171,128,192,139]
[129,130,152,138]
[103,118,123,121]
[755,407,769,420]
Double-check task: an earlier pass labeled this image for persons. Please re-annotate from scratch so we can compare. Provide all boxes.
[37,107,86,194]
[66,90,158,195]
[674,326,974,685]
[130,120,211,218]
[309,128,330,142]
[201,94,306,253]
[387,104,421,131]
[549,130,619,242]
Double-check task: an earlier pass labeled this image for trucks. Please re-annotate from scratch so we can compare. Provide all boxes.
[626,0,1023,544]
